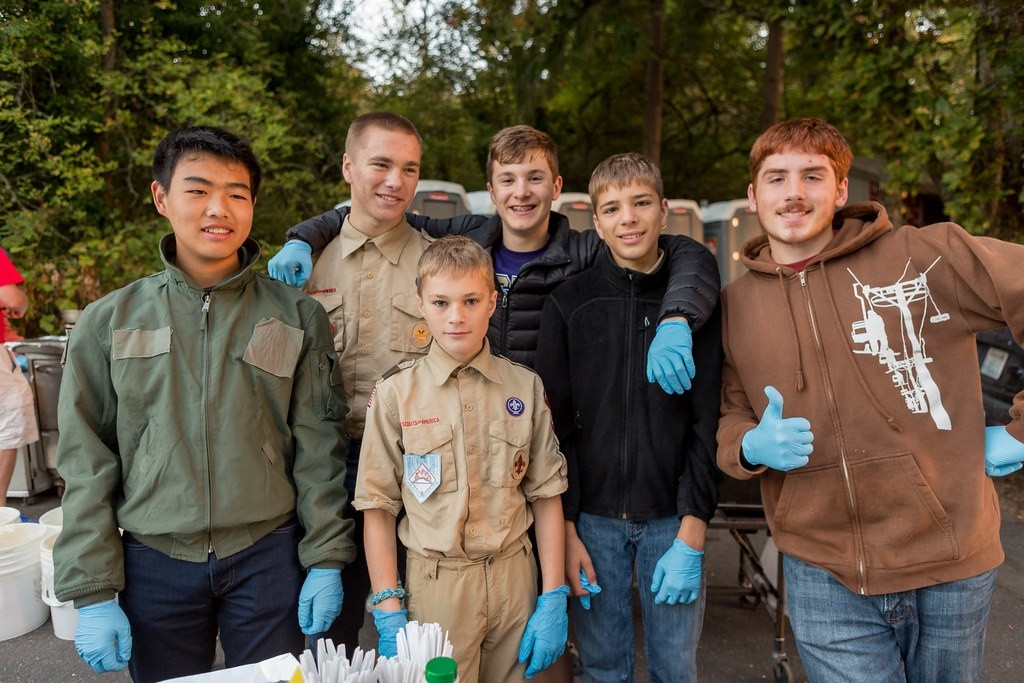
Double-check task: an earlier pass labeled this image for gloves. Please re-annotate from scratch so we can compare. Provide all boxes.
[268,240,314,288]
[741,386,814,471]
[646,321,696,394]
[650,539,705,604]
[518,585,569,679]
[298,568,345,634]
[373,608,409,659]
[985,425,1024,477]
[74,598,132,673]
[578,568,602,610]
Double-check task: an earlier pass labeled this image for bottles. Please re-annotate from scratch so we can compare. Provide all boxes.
[424,656,458,683]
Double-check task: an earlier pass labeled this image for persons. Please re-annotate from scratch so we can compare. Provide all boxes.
[266,110,1024,682]
[1,240,42,525]
[52,122,356,683]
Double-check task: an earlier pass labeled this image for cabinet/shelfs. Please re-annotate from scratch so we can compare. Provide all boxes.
[6,335,67,498]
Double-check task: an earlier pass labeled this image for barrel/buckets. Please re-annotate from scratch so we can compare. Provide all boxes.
[0,507,22,524]
[0,524,49,641]
[40,532,79,640]
[39,506,64,534]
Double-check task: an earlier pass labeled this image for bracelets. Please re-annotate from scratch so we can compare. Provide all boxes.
[371,587,406,606]
